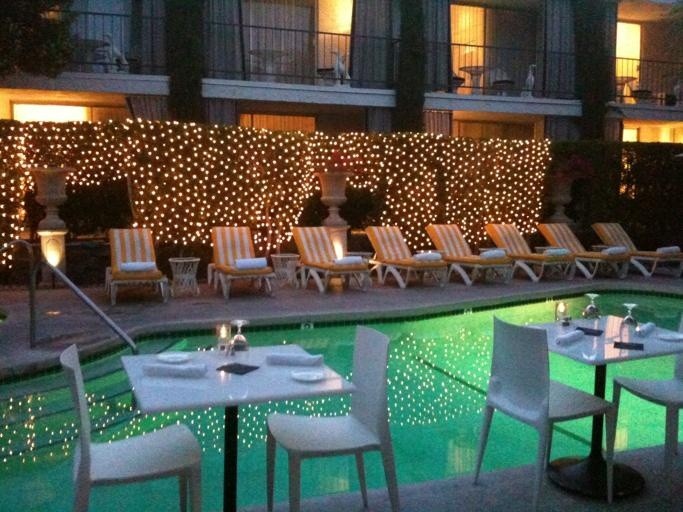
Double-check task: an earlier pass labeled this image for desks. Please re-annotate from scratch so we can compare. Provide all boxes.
[248,48,290,83]
[70,37,110,73]
[458,64,494,97]
[615,75,638,105]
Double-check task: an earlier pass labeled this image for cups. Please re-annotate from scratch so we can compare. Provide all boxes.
[215,324,231,356]
[554,302,568,322]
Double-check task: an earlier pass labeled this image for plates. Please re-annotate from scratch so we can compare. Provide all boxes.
[290,367,329,382]
[155,352,192,363]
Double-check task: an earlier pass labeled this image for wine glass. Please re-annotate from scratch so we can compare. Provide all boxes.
[231,319,248,352]
[620,303,639,329]
[584,293,600,320]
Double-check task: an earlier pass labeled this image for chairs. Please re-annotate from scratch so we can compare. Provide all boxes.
[266,323,402,512]
[473,316,616,512]
[58,344,203,512]
[610,313,683,504]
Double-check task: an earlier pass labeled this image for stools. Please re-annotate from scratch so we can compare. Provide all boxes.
[451,76,466,94]
[317,67,335,86]
[631,88,651,106]
[493,79,516,97]
